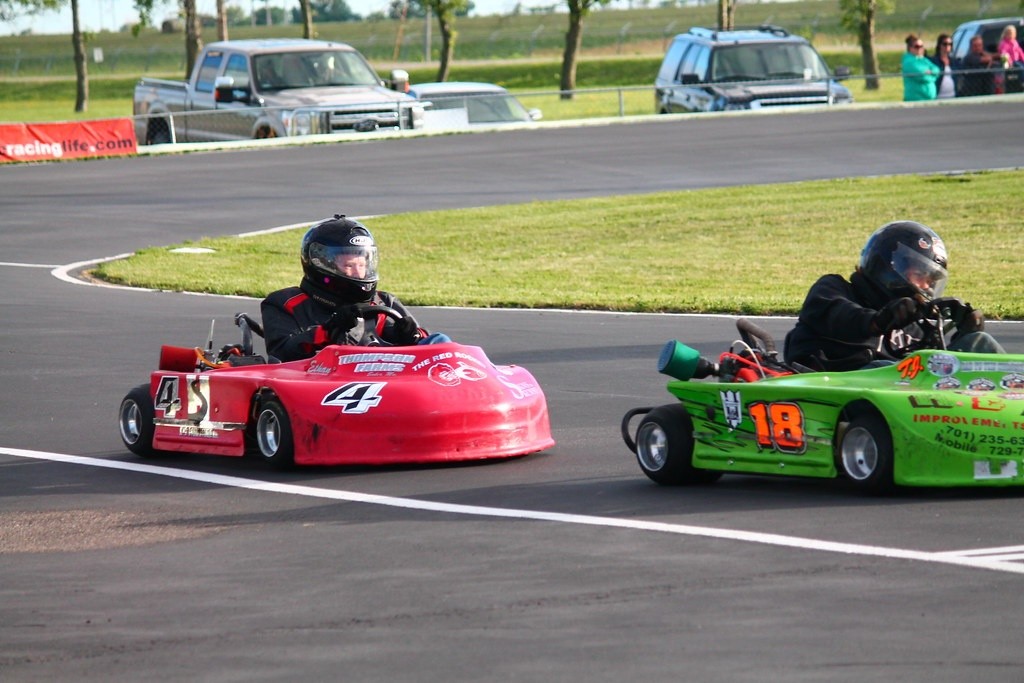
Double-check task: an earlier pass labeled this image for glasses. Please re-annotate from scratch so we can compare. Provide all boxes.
[942,43,952,46]
[913,45,923,49]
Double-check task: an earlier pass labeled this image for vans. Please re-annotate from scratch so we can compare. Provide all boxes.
[941,17,1024,97]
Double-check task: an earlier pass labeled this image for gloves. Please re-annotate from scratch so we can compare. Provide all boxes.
[875,296,917,330]
[323,305,360,341]
[956,309,984,337]
[394,316,417,344]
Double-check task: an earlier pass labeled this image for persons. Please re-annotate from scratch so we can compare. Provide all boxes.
[998,25,1024,67]
[961,36,1004,95]
[260,215,450,364]
[902,35,940,101]
[784,221,1005,375]
[926,34,960,97]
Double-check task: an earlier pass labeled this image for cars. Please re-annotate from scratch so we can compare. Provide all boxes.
[407,80,543,135]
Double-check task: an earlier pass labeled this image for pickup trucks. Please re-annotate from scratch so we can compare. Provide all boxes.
[133,37,423,148]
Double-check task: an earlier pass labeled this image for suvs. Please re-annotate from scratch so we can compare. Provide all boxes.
[654,26,852,118]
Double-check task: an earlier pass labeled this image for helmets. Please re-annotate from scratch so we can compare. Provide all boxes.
[860,221,948,308]
[301,213,379,301]
